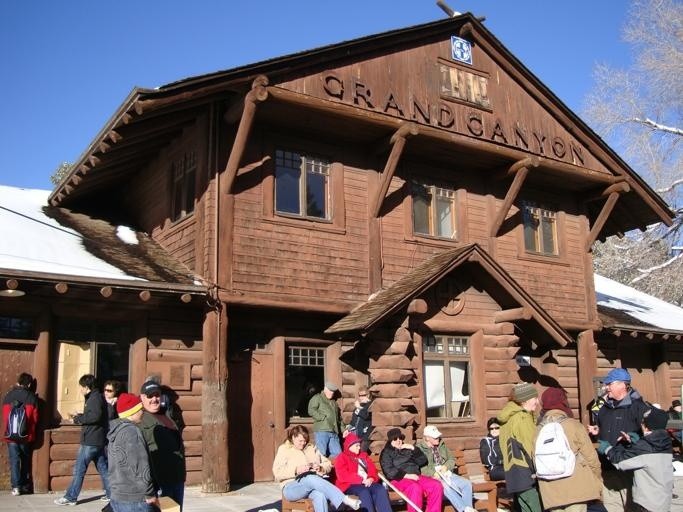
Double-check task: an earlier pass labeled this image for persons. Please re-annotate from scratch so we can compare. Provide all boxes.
[53,374,113,506]
[101,374,187,512]
[2,372,38,496]
[497,384,543,512]
[601,408,673,511]
[480,417,506,479]
[336,434,391,511]
[416,423,477,512]
[307,383,344,455]
[585,368,648,512]
[272,425,361,512]
[533,387,602,511]
[350,386,373,455]
[668,398,683,422]
[380,427,443,512]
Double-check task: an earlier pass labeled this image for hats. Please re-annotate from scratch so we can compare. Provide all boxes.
[643,406,670,430]
[386,428,405,444]
[116,392,144,419]
[423,425,443,439]
[672,399,681,407]
[140,380,162,395]
[599,368,631,384]
[487,418,501,428]
[508,381,539,403]
[326,383,338,392]
[541,386,574,417]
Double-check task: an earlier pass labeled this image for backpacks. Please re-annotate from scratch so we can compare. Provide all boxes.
[3,404,30,442]
[533,421,577,482]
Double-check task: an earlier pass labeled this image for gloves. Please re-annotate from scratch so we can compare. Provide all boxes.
[596,438,610,456]
[626,430,640,445]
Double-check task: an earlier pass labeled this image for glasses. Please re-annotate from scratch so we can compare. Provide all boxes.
[489,427,501,431]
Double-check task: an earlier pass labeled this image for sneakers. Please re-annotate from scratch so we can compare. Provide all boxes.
[11,487,21,495]
[347,498,362,511]
[100,495,112,502]
[53,497,78,506]
